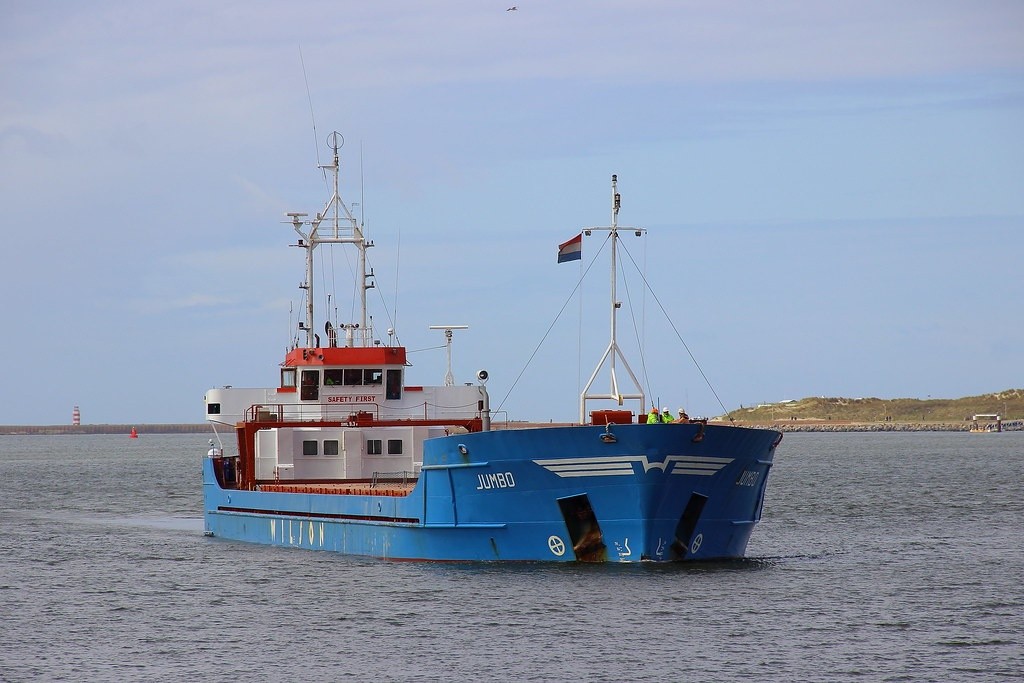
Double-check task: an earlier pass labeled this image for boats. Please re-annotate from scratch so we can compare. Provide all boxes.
[199,130,784,571]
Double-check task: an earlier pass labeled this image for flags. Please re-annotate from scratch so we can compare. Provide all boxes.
[557,234,581,264]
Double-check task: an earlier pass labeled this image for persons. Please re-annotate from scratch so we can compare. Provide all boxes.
[646,407,689,424]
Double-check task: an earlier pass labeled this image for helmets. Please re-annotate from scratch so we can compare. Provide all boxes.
[678,408,685,413]
[652,408,658,413]
[663,407,668,412]
